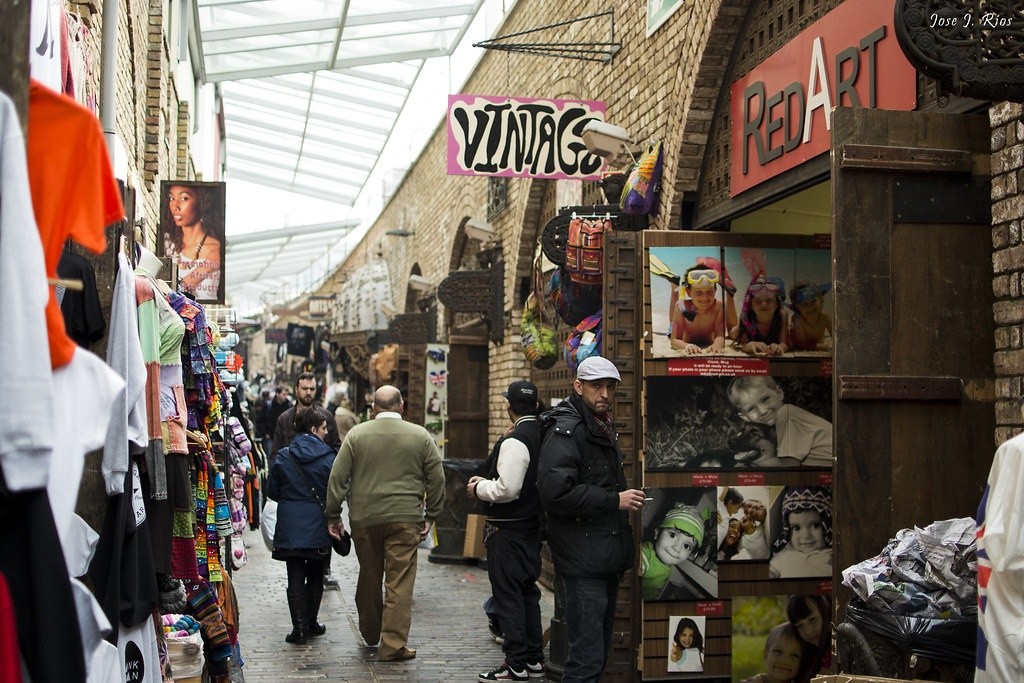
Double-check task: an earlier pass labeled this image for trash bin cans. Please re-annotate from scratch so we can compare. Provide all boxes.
[840,597,976,683]
[426,460,491,564]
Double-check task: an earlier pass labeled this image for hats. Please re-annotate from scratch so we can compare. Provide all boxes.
[502,381,537,403]
[773,486,834,555]
[576,356,622,383]
[659,502,711,549]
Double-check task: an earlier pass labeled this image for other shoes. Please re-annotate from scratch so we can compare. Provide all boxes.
[324,575,338,589]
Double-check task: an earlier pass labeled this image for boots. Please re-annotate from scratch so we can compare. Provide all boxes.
[285,588,326,645]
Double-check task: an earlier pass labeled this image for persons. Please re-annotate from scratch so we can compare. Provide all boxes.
[466,380,548,683]
[250,373,362,585]
[536,356,646,683]
[637,502,712,600]
[738,593,833,683]
[659,264,832,356]
[163,185,221,300]
[725,374,833,468]
[769,484,833,578]
[326,384,445,662]
[670,618,704,671]
[268,406,339,646]
[717,486,770,561]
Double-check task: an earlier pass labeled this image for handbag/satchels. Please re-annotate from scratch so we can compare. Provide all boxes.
[616,139,665,216]
[220,538,248,571]
[261,498,280,551]
[329,526,352,557]
[521,242,560,369]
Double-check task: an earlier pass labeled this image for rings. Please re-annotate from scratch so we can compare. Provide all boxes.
[637,502,640,506]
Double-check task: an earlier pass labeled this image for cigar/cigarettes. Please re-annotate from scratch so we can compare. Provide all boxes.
[645,498,654,501]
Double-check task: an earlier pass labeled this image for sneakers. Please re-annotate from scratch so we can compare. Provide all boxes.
[478,659,545,683]
[488,615,506,643]
[397,647,416,659]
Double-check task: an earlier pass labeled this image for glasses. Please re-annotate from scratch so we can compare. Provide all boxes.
[748,282,780,292]
[686,270,720,285]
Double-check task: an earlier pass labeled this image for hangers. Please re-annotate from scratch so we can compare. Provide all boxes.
[156,278,171,294]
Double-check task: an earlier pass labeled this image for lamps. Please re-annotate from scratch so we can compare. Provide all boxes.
[409,273,439,295]
[578,119,649,167]
[306,294,336,316]
[465,219,502,247]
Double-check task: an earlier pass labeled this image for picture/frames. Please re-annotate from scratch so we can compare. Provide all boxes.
[154,180,228,304]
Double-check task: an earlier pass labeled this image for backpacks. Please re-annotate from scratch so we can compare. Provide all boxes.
[563,309,606,370]
[547,267,603,325]
[566,216,617,288]
